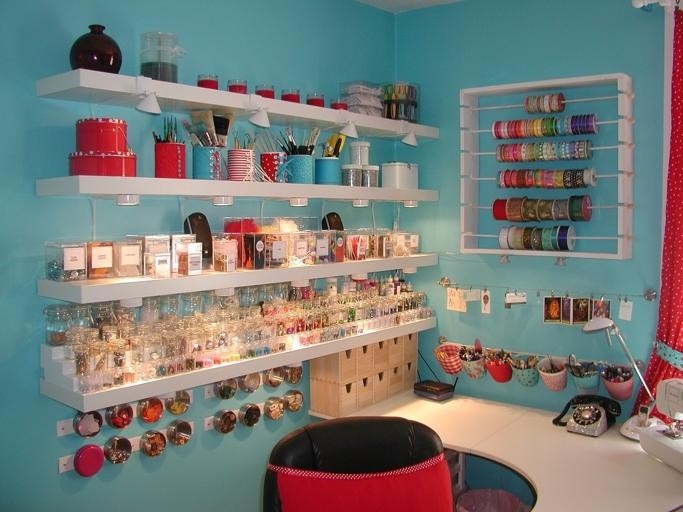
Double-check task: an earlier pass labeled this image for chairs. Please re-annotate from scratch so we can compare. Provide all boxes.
[263,417,453,512]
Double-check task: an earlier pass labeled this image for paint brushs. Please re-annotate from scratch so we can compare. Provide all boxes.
[276,127,321,156]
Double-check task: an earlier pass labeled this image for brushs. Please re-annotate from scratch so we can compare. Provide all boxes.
[331,138,342,158]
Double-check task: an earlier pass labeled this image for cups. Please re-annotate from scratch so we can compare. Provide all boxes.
[196,74,348,109]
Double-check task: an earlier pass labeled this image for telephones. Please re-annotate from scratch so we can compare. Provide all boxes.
[621,404,663,442]
[566,394,621,437]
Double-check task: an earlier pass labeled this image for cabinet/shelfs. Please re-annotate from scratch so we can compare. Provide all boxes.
[309,332,418,418]
[35,69,440,414]
[457,73,635,262]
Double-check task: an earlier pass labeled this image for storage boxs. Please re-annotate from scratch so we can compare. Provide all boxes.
[382,161,419,190]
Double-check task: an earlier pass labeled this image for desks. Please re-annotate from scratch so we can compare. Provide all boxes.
[308,387,683,512]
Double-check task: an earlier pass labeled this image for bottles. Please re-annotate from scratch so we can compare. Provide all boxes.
[42,272,434,394]
[341,141,379,187]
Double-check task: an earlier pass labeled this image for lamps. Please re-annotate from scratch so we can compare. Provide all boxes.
[584,317,664,441]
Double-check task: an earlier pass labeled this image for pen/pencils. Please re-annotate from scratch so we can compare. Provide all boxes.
[153,114,177,144]
[234,130,257,149]
[182,119,216,147]
[322,144,334,157]
